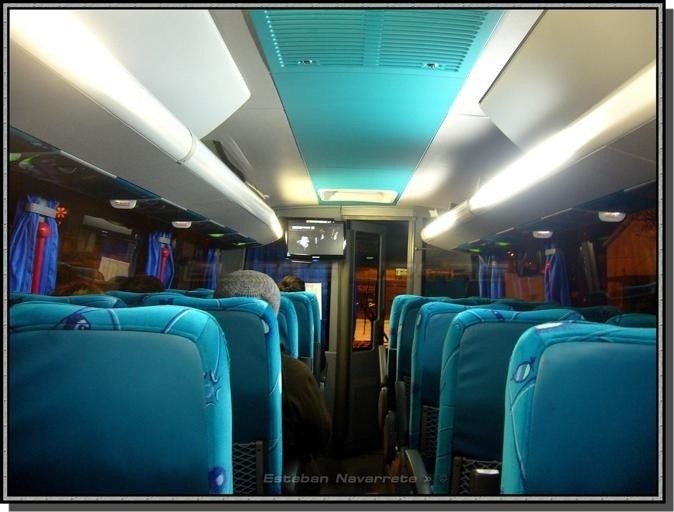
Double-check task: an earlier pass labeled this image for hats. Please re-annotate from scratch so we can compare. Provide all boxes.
[214,268,283,335]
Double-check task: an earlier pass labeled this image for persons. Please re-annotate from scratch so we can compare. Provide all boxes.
[119,275,166,293]
[210,267,335,460]
[275,272,326,374]
[49,279,103,299]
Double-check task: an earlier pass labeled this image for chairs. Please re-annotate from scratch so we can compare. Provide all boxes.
[376,293,659,497]
[5,287,321,499]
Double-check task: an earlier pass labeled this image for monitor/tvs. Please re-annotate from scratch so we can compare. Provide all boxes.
[286,219,347,262]
